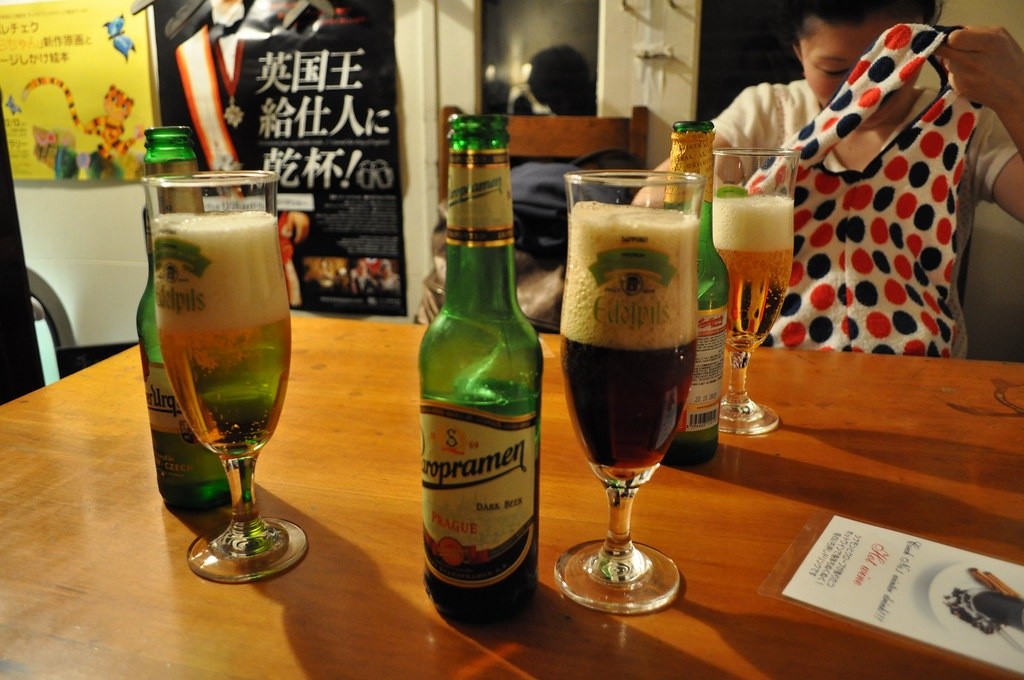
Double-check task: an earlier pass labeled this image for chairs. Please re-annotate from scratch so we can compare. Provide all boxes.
[417,105,652,335]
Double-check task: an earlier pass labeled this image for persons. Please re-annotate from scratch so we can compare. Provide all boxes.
[353,258,401,297]
[631,0,1024,358]
[159,0,318,311]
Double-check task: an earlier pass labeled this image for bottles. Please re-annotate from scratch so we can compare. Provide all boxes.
[418,114,544,630]
[659,120,730,469]
[136,126,253,511]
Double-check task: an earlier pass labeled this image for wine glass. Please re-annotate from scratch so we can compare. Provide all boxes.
[553,169,704,615]
[713,146,803,435]
[141,168,309,583]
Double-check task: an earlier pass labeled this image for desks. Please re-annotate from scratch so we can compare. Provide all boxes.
[0,313,1024,680]
